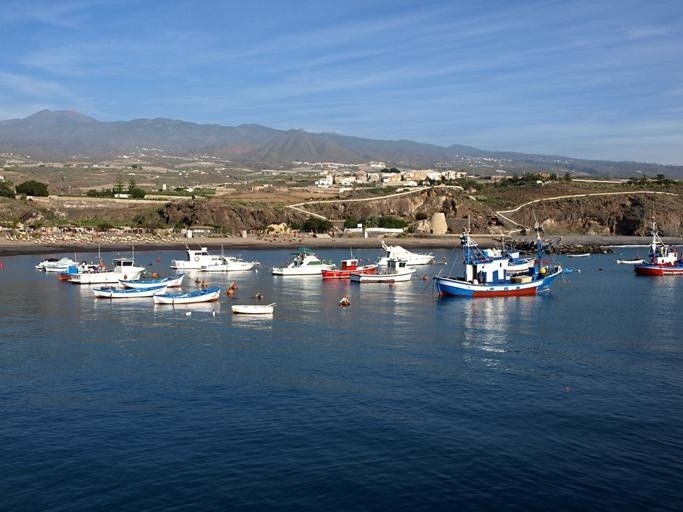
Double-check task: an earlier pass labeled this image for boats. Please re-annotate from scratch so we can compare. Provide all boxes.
[271,246,336,276]
[230,303,277,315]
[429,206,563,299]
[634,216,683,276]
[616,258,644,265]
[567,252,591,258]
[320,239,448,284]
[33,241,261,305]
